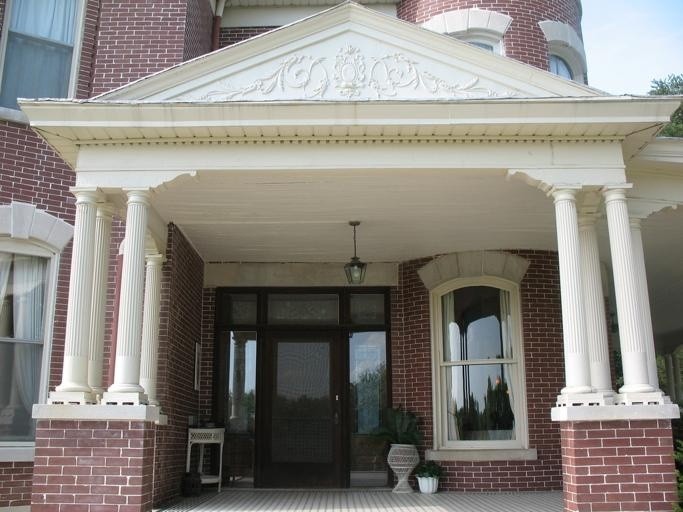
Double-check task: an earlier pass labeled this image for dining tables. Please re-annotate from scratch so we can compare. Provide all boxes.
[370,404,424,494]
[413,460,442,494]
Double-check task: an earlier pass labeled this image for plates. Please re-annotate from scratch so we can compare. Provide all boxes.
[186,428,225,492]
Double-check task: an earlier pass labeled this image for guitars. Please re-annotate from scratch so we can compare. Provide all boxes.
[344,256,367,284]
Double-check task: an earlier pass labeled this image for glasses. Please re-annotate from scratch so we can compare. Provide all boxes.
[343,220,367,285]
